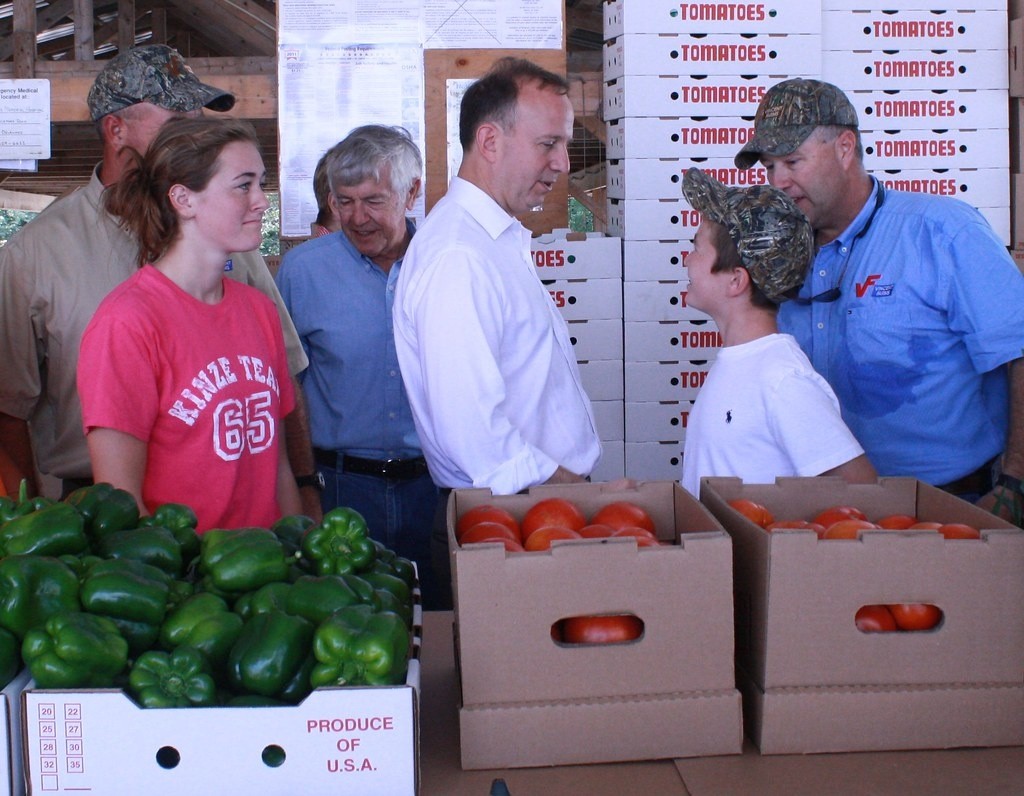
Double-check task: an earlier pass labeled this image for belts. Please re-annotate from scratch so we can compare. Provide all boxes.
[313,447,426,480]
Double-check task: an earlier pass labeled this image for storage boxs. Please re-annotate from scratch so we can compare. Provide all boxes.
[0,665,31,796]
[445,479,744,771]
[700,474,1023,754]
[18,560,423,796]
[530,0,1012,490]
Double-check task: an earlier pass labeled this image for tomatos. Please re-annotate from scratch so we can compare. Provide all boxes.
[456,498,979,643]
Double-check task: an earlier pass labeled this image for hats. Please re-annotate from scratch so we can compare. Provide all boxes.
[86,43,236,121]
[681,166,815,307]
[734,77,858,170]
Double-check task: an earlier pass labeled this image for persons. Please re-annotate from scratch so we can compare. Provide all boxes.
[268,124,453,610]
[733,74,1024,533]
[677,166,880,486]
[391,57,603,610]
[76,113,304,534]
[0,43,327,532]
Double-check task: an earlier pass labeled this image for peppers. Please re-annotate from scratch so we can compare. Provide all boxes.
[0,477,416,708]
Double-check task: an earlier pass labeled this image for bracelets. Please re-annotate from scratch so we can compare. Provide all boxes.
[995,471,1024,495]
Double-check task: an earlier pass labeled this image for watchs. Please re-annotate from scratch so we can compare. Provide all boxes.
[291,471,326,490]
